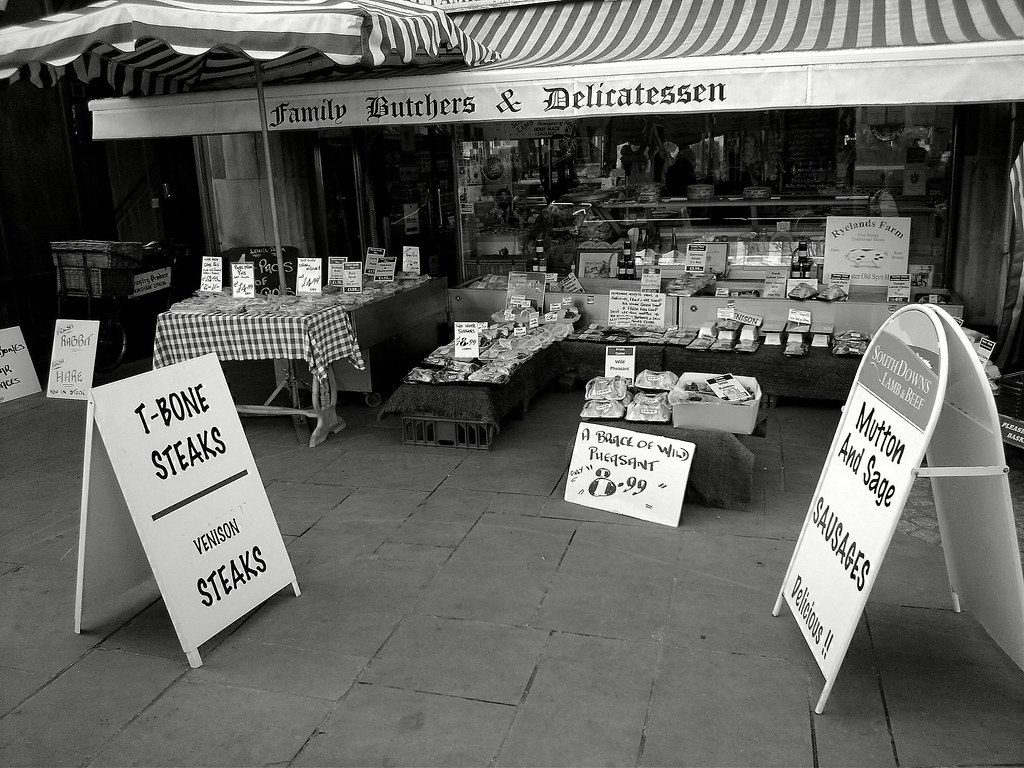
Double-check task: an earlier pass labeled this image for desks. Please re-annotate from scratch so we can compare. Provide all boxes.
[149,275,447,448]
[376,330,866,452]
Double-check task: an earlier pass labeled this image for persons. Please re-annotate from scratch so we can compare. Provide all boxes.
[539,133,579,205]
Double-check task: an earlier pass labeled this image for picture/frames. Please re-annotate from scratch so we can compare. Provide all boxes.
[575,248,623,279]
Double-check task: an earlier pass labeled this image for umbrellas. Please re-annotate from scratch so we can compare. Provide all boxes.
[0,0,501,409]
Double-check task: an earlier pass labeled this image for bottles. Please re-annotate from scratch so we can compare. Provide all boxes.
[671,228,677,250]
[618,241,635,280]
[758,228,769,242]
[791,242,811,278]
[531,239,549,272]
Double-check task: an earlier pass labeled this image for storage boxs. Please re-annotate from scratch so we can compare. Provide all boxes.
[668,371,762,434]
[475,228,533,257]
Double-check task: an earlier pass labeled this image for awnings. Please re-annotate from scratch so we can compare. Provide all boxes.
[85,0,1023,139]
